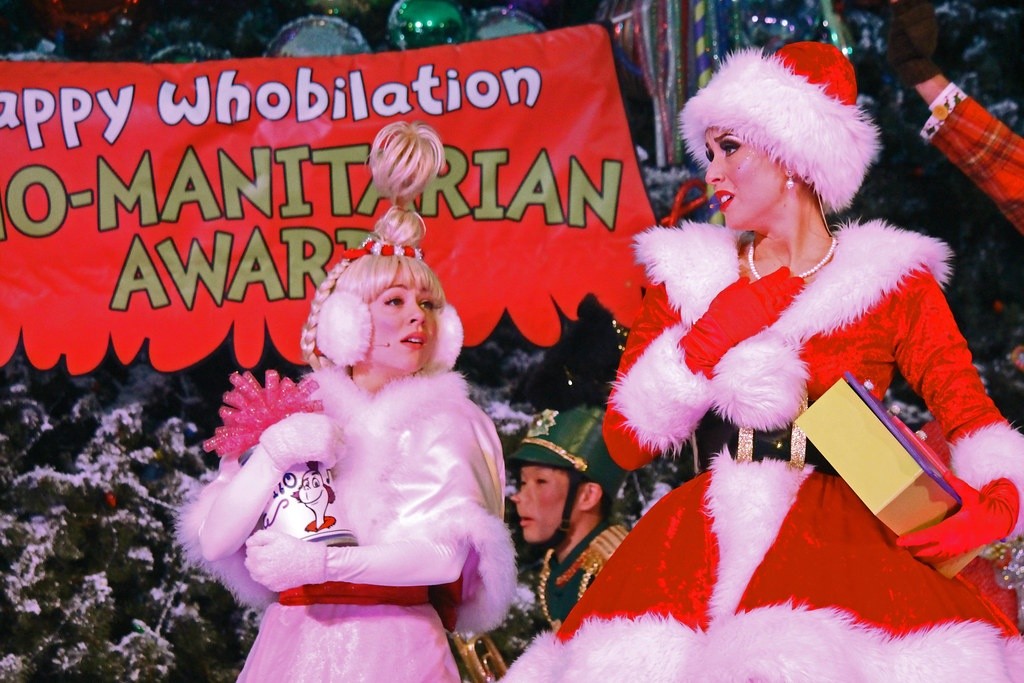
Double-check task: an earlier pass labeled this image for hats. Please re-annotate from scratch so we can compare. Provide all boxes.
[506,406,633,503]
[678,40,880,213]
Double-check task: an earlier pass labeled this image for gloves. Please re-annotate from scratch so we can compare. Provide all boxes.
[896,470,1020,563]
[676,265,806,379]
[886,0,942,88]
[244,529,328,592]
[259,413,346,470]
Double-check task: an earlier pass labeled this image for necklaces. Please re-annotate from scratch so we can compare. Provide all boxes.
[748,236,836,280]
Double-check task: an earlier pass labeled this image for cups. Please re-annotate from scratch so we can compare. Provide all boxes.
[237,443,359,546]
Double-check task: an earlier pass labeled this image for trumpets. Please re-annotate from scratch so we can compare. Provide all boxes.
[452,632,508,682]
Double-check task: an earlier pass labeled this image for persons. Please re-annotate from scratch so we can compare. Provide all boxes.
[175,120,519,682]
[884,0,1024,233]
[509,402,632,633]
[502,41,1024,683]
